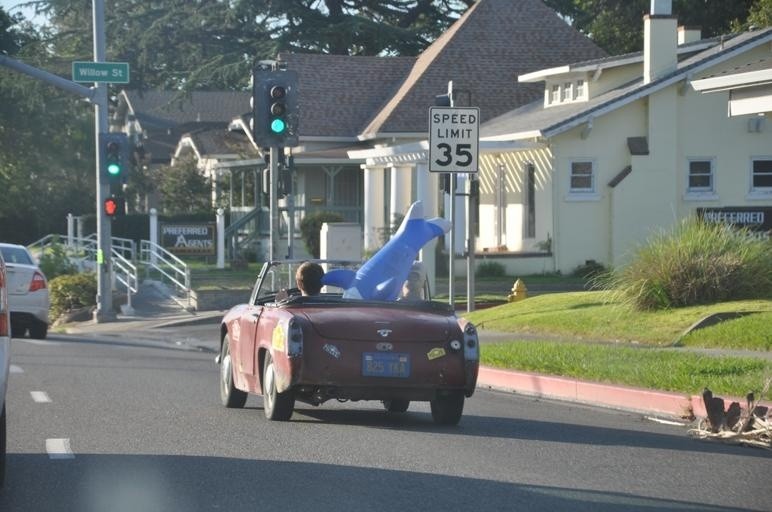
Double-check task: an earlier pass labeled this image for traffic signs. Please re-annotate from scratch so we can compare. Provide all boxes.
[429,106,480,173]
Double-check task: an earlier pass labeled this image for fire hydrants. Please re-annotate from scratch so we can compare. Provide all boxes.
[509,278,526,300]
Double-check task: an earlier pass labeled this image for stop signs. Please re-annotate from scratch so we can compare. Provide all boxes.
[104,197,116,215]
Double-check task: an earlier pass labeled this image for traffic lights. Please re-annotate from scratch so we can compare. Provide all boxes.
[99,132,130,185]
[435,95,450,106]
[253,68,300,148]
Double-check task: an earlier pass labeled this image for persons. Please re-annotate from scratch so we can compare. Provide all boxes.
[275,261,325,303]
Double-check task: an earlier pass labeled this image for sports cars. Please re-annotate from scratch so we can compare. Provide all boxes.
[215,259,479,424]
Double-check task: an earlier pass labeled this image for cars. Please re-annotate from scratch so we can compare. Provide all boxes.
[0,243,49,415]
